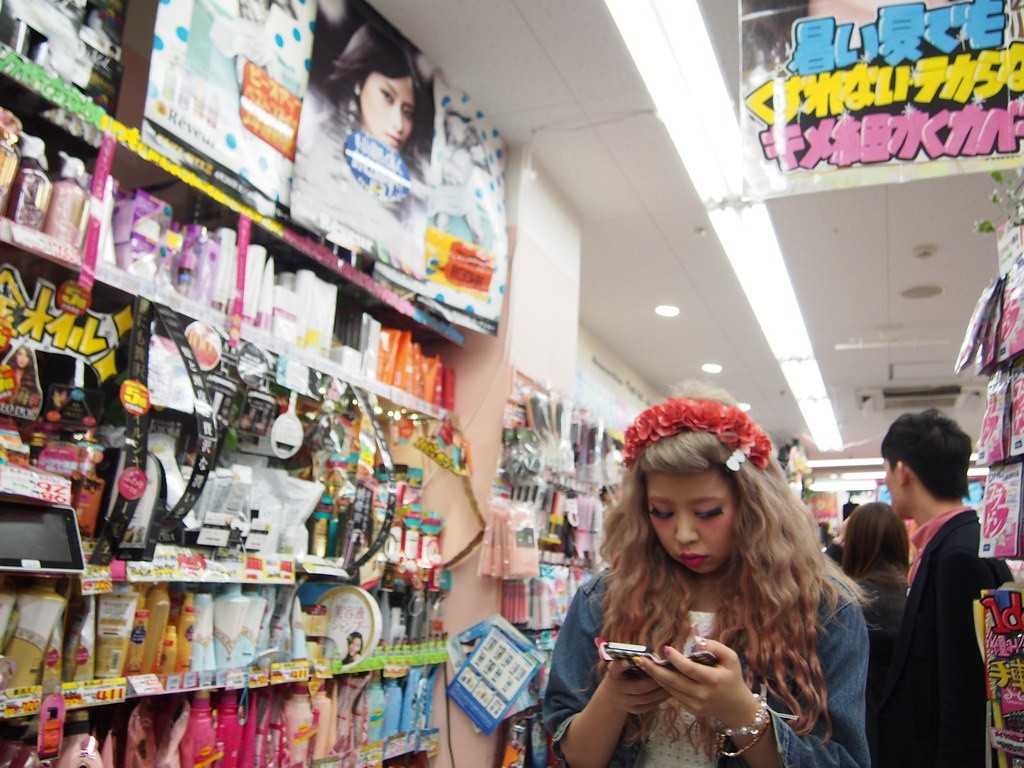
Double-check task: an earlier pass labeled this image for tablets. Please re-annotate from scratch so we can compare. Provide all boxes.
[0,500,86,574]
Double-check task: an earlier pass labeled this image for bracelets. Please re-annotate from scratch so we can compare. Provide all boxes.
[712,693,771,758]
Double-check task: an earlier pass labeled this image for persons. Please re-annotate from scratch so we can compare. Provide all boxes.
[290,23,429,269]
[541,386,872,768]
[874,408,1015,768]
[824,513,852,566]
[844,501,909,728]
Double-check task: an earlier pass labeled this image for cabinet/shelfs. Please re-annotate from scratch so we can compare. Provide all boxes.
[0,42,466,768]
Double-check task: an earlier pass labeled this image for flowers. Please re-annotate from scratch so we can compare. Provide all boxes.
[623,399,772,471]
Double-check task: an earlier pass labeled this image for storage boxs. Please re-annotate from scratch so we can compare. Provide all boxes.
[72,478,106,536]
[331,345,362,370]
[113,189,173,273]
[308,278,338,352]
[195,464,252,522]
[115,1,320,217]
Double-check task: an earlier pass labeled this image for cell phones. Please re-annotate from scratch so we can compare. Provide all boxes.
[608,641,661,661]
